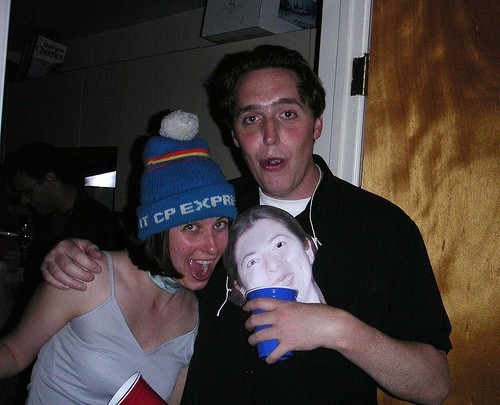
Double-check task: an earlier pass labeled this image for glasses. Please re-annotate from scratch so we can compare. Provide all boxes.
[8,177,43,199]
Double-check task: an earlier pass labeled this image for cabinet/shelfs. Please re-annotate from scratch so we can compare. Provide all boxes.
[0,27,316,211]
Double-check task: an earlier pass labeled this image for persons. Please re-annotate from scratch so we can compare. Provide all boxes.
[40,44,453,405]
[0,109,239,405]
[223,204,328,308]
[0,140,123,332]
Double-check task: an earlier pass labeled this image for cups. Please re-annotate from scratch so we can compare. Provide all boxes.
[246,286,299,360]
[107,371,169,405]
[0,232,21,263]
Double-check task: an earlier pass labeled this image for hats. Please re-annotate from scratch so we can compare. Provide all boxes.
[137,110,236,241]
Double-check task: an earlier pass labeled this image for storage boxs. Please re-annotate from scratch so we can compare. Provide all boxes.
[201,0,317,44]
